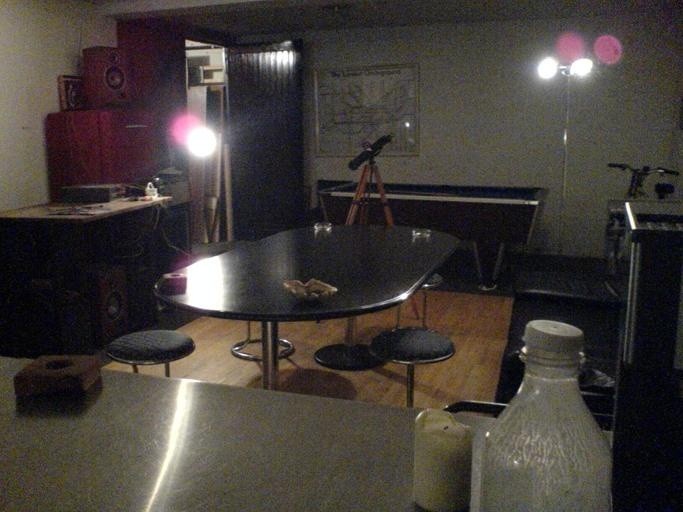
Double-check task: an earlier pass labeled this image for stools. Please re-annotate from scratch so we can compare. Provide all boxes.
[230,320,295,361]
[106,330,195,378]
[369,324,455,409]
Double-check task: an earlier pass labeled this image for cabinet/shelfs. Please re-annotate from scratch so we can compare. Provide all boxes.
[452,200,682,512]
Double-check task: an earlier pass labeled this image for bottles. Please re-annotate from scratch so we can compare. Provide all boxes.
[481,317,612,510]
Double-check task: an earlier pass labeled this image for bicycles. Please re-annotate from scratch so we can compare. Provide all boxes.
[609,162,679,200]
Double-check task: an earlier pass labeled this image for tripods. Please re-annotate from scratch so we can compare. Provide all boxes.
[311,165,421,324]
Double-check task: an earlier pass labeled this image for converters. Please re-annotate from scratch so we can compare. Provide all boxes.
[145,187,158,197]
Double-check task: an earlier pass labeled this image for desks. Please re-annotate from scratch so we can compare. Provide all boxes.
[0,196,173,366]
[151,222,459,393]
[0,353,611,512]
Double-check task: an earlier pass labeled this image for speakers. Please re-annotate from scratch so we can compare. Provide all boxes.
[97,266,128,337]
[81,45,135,106]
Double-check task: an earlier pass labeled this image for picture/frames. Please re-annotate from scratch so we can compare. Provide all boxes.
[58,75,87,112]
[315,64,420,158]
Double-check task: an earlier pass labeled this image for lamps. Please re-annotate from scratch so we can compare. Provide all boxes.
[539,58,592,257]
